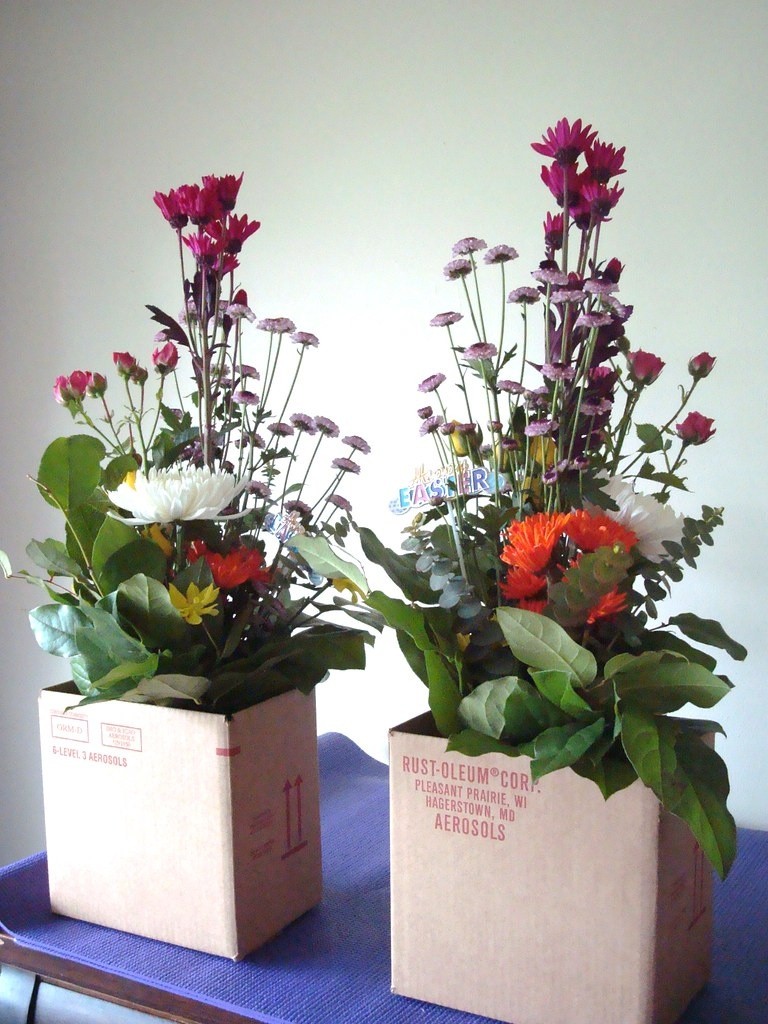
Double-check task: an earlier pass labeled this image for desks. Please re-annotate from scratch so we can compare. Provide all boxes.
[0,729,768,1024]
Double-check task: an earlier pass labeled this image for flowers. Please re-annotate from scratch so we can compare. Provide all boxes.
[351,111,748,881]
[0,171,384,717]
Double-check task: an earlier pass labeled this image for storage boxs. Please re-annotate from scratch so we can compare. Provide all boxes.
[386,709,712,1024]
[35,681,324,962]
